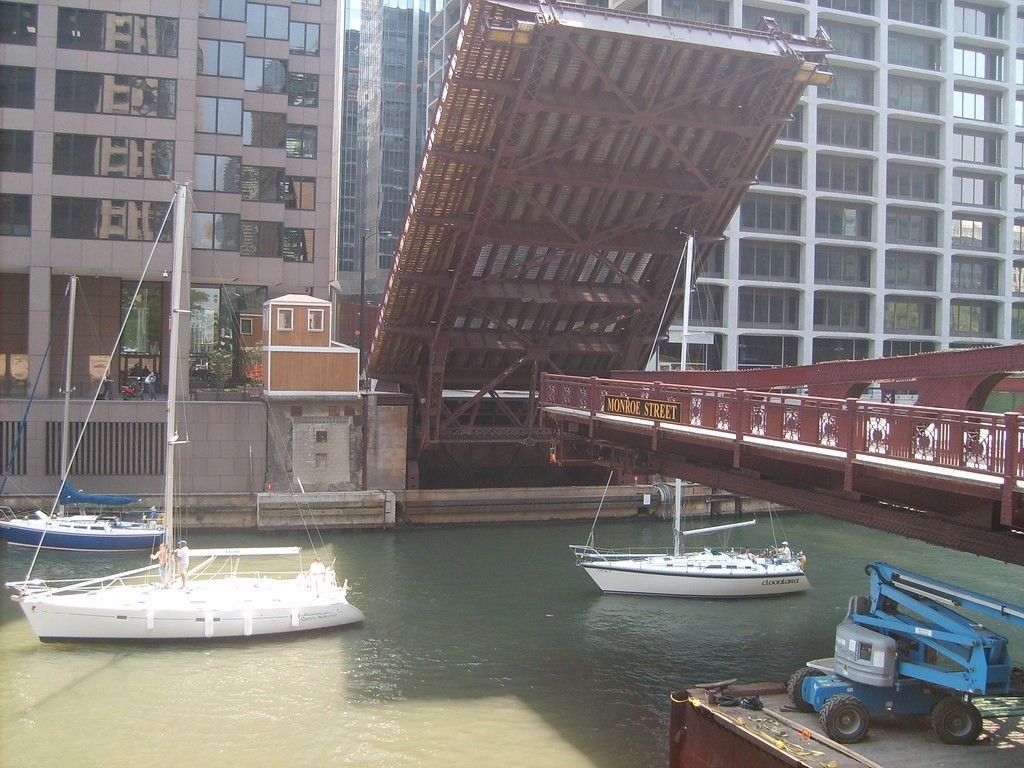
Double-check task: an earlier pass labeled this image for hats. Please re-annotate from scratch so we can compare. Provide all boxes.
[178,540,187,544]
[781,541,788,546]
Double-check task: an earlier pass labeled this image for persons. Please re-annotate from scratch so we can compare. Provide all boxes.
[772,541,791,565]
[102,370,114,400]
[150,544,166,580]
[143,365,151,376]
[743,547,757,564]
[144,371,156,401]
[174,540,189,589]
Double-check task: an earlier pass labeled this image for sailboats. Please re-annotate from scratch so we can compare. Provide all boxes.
[0,274,165,556]
[4,177,365,644]
[569,226,812,599]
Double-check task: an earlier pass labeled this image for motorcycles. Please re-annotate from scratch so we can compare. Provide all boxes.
[121,377,145,401]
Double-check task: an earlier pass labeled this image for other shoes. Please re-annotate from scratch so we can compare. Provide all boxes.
[109,398,114,400]
[179,587,185,589]
[101,398,105,400]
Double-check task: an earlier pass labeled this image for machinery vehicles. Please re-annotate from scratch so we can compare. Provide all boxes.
[786,560,1023,743]
